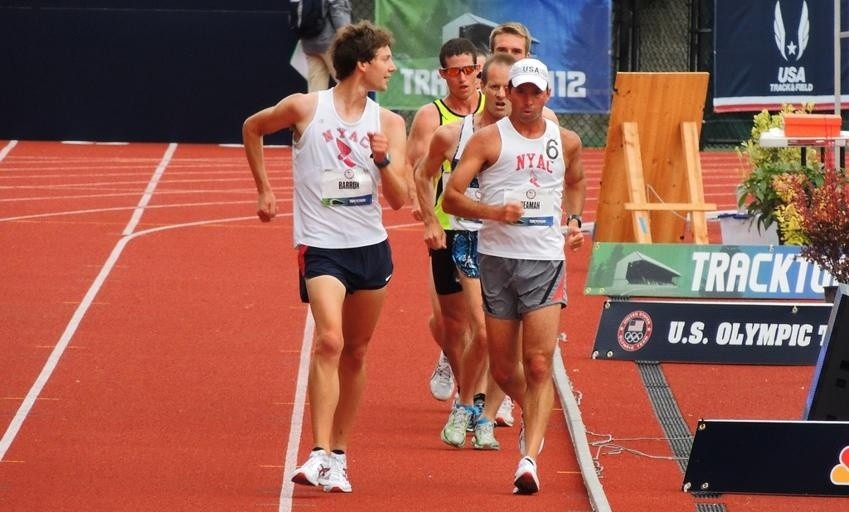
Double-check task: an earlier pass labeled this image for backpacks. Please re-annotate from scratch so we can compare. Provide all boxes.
[299,0,327,38]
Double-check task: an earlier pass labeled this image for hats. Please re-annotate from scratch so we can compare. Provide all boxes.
[508,58,549,91]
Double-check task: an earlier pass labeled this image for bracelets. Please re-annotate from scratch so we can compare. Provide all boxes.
[566,211,585,227]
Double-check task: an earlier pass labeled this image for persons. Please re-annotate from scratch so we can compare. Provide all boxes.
[408,38,499,433]
[243,18,411,494]
[430,19,559,427]
[414,51,519,453]
[293,2,354,93]
[443,56,586,493]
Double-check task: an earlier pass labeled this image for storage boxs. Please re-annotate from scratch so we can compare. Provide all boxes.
[783,114,843,137]
[718,212,780,245]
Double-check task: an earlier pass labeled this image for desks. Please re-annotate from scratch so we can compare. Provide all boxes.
[758,128,849,175]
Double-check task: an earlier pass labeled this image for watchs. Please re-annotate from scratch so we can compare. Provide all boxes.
[369,151,393,167]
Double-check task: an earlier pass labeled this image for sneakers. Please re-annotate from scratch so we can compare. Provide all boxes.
[428,349,547,456]
[291,448,354,495]
[511,457,542,496]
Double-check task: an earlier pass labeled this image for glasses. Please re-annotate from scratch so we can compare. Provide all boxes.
[441,64,479,76]
